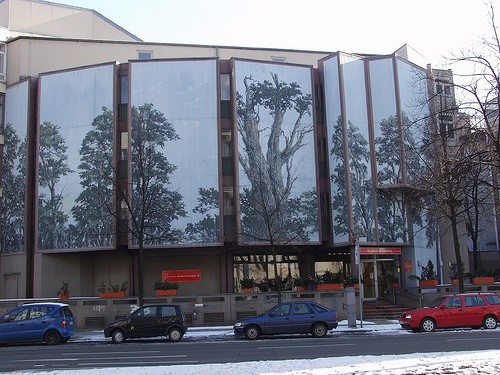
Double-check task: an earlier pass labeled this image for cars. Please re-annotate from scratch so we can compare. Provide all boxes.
[103,303,188,344]
[399,291,500,332]
[0,302,76,345]
[233,301,338,340]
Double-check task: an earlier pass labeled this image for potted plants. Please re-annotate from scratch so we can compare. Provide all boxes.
[98,280,128,298]
[309,271,345,291]
[408,265,438,287]
[351,278,362,288]
[57,282,70,300]
[294,278,306,291]
[240,278,254,293]
[154,281,178,296]
[392,278,400,288]
[449,266,465,286]
[470,270,494,285]
